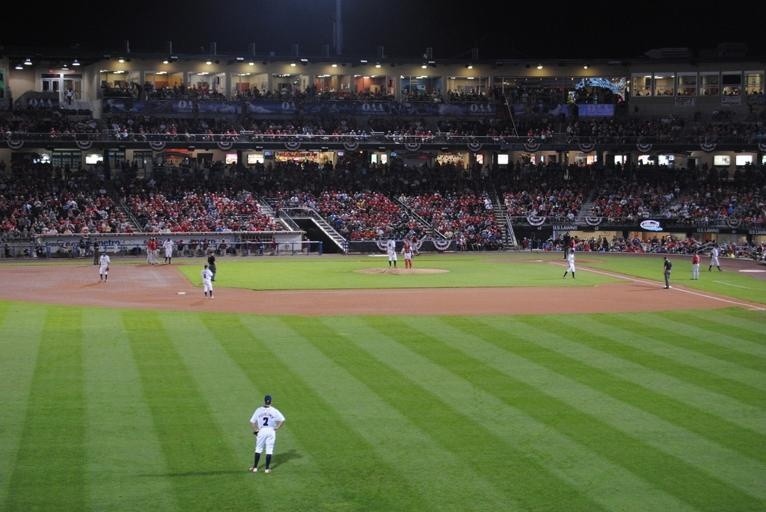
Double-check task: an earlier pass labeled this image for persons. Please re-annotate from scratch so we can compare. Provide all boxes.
[199,265,214,299]
[0,74,766,270]
[561,249,576,280]
[662,256,672,289]
[96,253,110,282]
[206,251,216,281]
[689,253,700,280]
[248,394,286,474]
[706,244,722,272]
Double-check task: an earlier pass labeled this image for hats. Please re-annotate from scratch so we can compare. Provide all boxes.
[265,395,271,402]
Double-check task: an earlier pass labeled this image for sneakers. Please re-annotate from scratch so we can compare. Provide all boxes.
[265,468,272,473]
[248,467,257,472]
